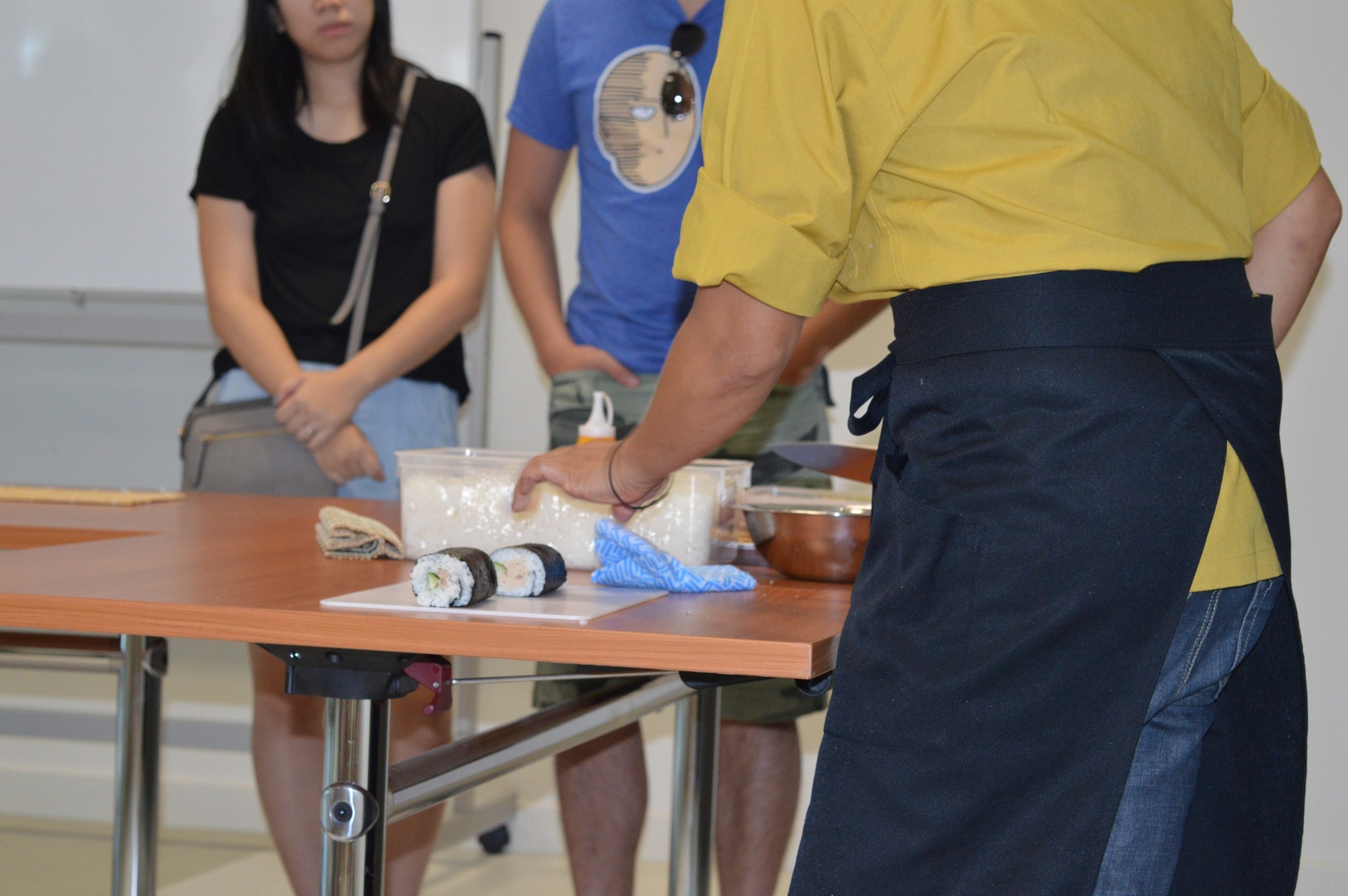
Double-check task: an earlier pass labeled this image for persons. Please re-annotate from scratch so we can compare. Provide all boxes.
[497,1,829,895]
[511,1,1342,896]
[189,2,500,896]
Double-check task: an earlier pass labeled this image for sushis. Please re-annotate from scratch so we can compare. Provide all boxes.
[410,547,498,608]
[489,544,566,597]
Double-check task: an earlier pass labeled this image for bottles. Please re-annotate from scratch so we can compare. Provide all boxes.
[577,390,616,447]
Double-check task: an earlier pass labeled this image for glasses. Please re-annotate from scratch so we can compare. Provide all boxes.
[661,20,706,117]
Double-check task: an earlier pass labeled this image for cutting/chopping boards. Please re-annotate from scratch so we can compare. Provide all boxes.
[321,579,668,621]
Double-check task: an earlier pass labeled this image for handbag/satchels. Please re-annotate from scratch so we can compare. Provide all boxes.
[180,396,339,499]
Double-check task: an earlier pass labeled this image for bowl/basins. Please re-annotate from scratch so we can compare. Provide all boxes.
[729,498,871,583]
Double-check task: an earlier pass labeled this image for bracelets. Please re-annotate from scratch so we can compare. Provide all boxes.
[609,434,675,509]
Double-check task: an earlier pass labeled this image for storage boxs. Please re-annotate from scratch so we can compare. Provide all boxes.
[396,445,751,573]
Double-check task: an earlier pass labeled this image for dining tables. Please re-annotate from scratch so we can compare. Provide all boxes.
[0,485,854,896]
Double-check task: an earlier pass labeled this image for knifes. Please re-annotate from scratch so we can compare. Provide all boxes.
[770,442,876,484]
